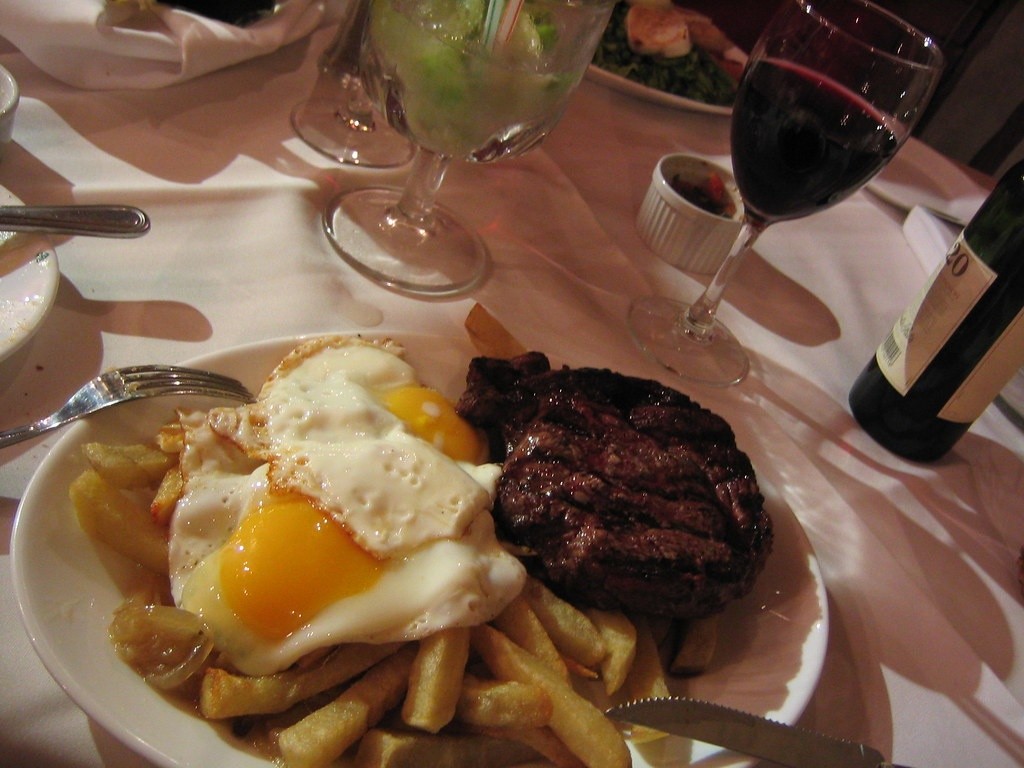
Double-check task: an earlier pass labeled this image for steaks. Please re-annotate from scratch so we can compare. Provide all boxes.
[453,348,774,621]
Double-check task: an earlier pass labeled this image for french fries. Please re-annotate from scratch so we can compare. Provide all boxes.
[69,303,721,768]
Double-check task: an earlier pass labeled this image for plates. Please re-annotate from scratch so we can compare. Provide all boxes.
[584,45,750,115]
[1,185,61,364]
[11,333,832,768]
[868,137,986,224]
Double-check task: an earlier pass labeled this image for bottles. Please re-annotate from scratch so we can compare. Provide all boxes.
[849,156,1024,465]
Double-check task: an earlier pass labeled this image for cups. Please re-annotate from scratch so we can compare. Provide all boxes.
[0,64,19,163]
[634,154,744,274]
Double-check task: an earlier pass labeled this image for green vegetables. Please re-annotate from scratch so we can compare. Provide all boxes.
[589,0,738,107]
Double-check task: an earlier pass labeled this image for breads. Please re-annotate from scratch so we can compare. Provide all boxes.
[625,0,691,58]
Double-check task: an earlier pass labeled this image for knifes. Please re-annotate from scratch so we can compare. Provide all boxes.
[604,696,915,768]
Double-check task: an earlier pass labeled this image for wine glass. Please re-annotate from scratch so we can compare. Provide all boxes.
[322,0,616,301]
[290,0,416,169]
[627,0,946,387]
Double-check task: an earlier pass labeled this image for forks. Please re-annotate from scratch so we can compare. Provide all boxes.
[0,366,257,449]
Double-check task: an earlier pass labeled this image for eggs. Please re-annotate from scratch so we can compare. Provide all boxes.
[206,331,507,558]
[164,406,527,674]
